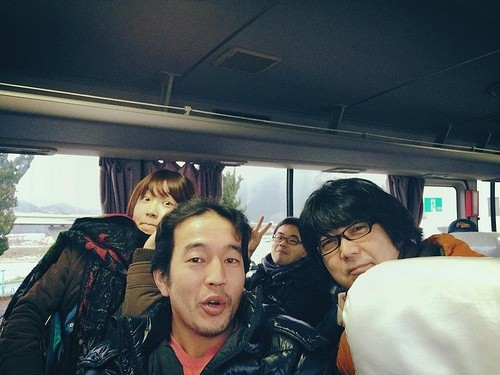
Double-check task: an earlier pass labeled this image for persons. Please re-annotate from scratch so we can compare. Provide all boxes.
[245,215,326,328]
[298,178,487,375]
[77,194,342,375]
[448,219,478,233]
[0,169,196,375]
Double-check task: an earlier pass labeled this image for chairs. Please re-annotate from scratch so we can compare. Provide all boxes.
[341,256,500,375]
[450,231,500,258]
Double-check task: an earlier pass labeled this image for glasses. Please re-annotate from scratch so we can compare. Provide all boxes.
[313,218,377,257]
[271,233,304,245]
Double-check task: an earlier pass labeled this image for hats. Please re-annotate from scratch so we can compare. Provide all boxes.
[448,219,478,233]
